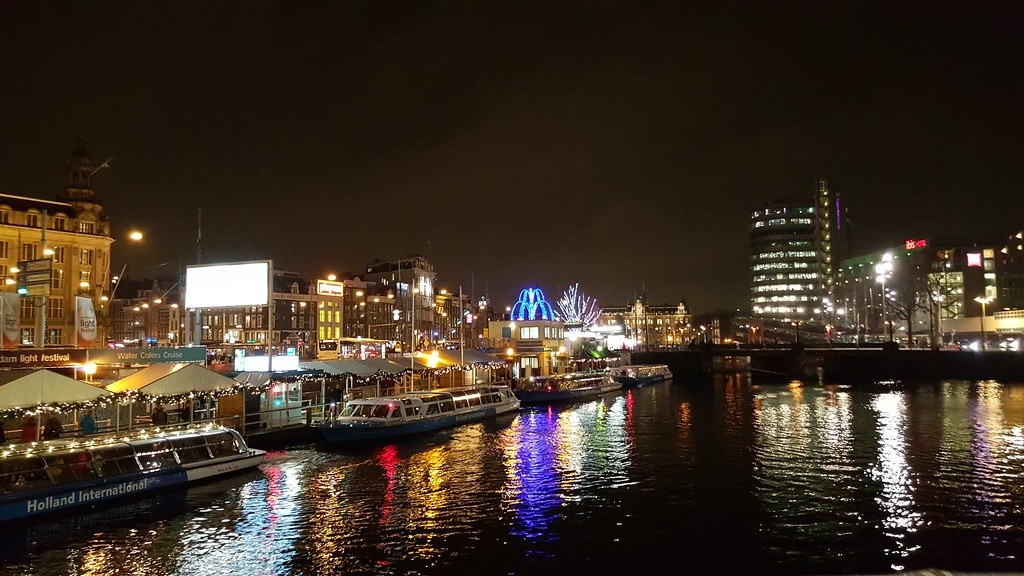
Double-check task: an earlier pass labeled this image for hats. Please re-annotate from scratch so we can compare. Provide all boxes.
[154,405,164,410]
[83,409,95,415]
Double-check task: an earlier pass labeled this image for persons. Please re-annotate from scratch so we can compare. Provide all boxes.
[151,402,169,426]
[77,409,98,437]
[320,384,344,417]
[21,414,37,442]
[43,410,63,440]
[831,333,969,348]
[181,400,197,427]
[0,417,9,445]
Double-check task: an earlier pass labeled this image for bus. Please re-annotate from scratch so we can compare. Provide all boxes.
[317,337,402,362]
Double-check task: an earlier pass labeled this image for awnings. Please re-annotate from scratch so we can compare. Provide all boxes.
[300,348,508,383]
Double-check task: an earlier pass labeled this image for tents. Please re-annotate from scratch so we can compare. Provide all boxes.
[569,342,621,371]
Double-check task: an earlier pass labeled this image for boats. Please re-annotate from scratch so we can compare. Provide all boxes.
[514,371,622,402]
[613,364,673,388]
[0,422,267,532]
[309,382,522,446]
